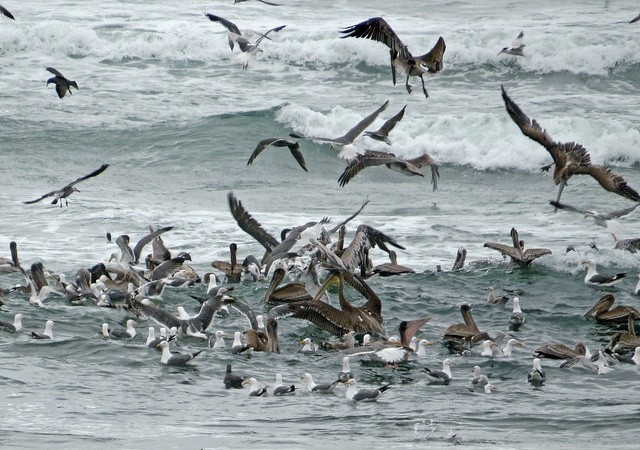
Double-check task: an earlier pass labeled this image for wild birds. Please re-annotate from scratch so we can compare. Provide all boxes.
[480,340,498,357]
[483,226,553,268]
[145,325,166,347]
[441,302,489,354]
[362,105,407,145]
[0,223,201,312]
[497,338,523,357]
[337,149,440,191]
[21,164,112,207]
[98,319,139,340]
[508,296,525,326]
[247,137,308,173]
[484,286,510,305]
[273,373,300,395]
[0,313,24,333]
[288,99,390,150]
[170,325,177,338]
[198,188,434,367]
[232,0,281,9]
[338,356,353,383]
[500,83,640,213]
[534,342,589,359]
[45,67,81,99]
[496,30,526,57]
[584,294,640,323]
[242,378,268,397]
[223,364,248,388]
[205,13,263,54]
[30,320,56,340]
[301,372,343,395]
[582,258,627,288]
[130,295,225,339]
[418,358,457,386]
[437,246,467,273]
[471,365,489,388]
[91,323,111,340]
[559,350,615,375]
[548,198,640,227]
[156,341,203,366]
[345,379,393,403]
[242,24,286,70]
[484,383,497,395]
[159,328,175,342]
[528,357,546,382]
[337,17,446,98]
[604,312,640,352]
